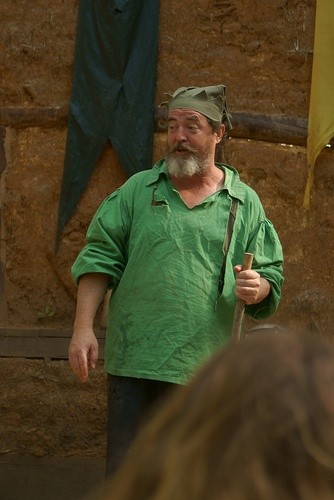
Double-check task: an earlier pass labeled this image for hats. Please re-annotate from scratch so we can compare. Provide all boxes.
[156,83,233,130]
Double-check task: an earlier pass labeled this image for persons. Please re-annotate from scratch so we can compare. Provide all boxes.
[81,327,334,500]
[67,84,286,500]
